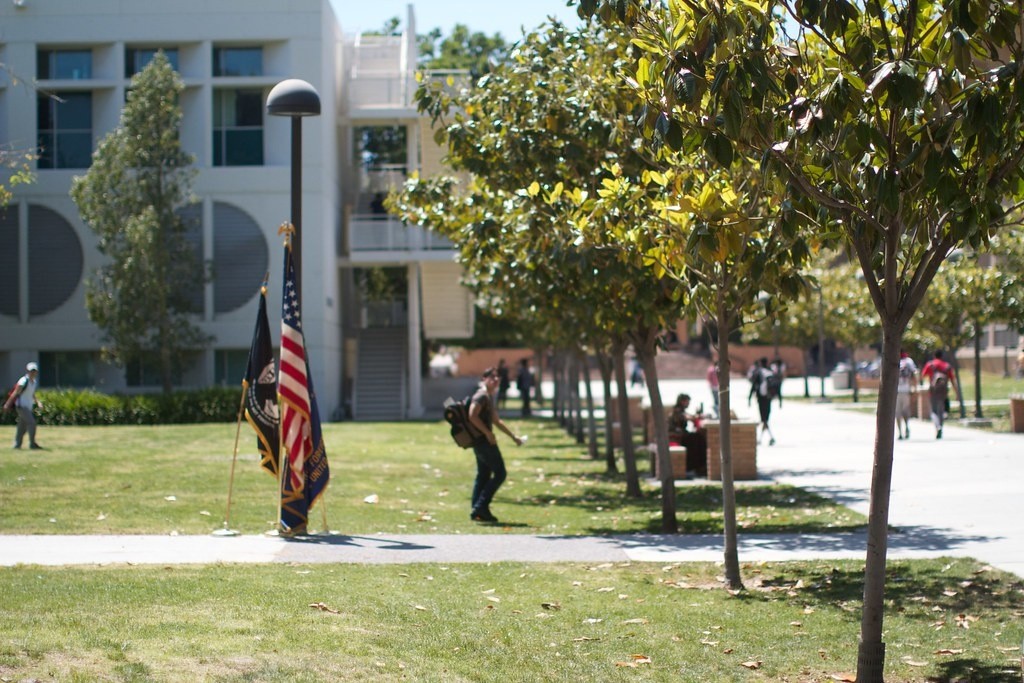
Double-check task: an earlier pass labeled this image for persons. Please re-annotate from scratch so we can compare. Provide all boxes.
[666,394,698,478]
[517,358,538,416]
[629,357,645,388]
[748,356,786,445]
[707,360,719,416]
[894,348,918,439]
[3,362,44,450]
[495,359,511,411]
[1013,345,1024,382]
[469,367,522,524]
[835,354,881,379]
[920,349,960,439]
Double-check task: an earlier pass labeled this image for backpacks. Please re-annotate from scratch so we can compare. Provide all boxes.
[9,375,27,402]
[758,367,778,399]
[929,362,950,397]
[443,393,484,449]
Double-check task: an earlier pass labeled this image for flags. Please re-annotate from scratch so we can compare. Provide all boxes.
[242,281,280,481]
[278,243,330,538]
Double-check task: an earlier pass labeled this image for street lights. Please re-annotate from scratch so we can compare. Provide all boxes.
[264,79,322,329]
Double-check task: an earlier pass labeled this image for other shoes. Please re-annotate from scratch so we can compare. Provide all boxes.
[472,509,499,523]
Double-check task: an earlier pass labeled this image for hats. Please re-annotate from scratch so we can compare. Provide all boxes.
[26,361,37,371]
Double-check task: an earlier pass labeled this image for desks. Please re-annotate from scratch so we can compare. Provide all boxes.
[700,419,759,480]
[613,396,642,426]
[644,405,676,444]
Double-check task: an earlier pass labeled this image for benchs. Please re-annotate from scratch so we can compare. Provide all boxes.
[612,422,633,446]
[647,444,687,479]
